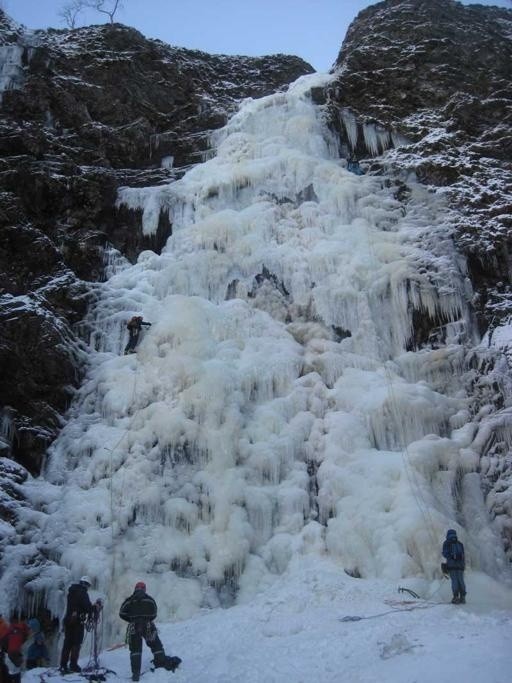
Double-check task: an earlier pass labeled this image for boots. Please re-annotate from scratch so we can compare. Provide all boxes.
[452,592,466,603]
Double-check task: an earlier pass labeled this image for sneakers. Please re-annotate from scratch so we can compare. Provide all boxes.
[129,348,137,353]
[59,667,74,674]
[71,665,81,672]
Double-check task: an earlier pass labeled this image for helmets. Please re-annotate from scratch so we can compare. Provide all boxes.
[35,633,44,640]
[135,582,146,593]
[28,618,40,632]
[80,576,92,585]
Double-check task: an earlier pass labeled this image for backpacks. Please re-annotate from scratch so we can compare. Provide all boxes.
[128,316,139,329]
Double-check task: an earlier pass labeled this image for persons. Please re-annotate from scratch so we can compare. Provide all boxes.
[119,582,166,681]
[442,529,467,603]
[59,576,98,670]
[0,609,58,682]
